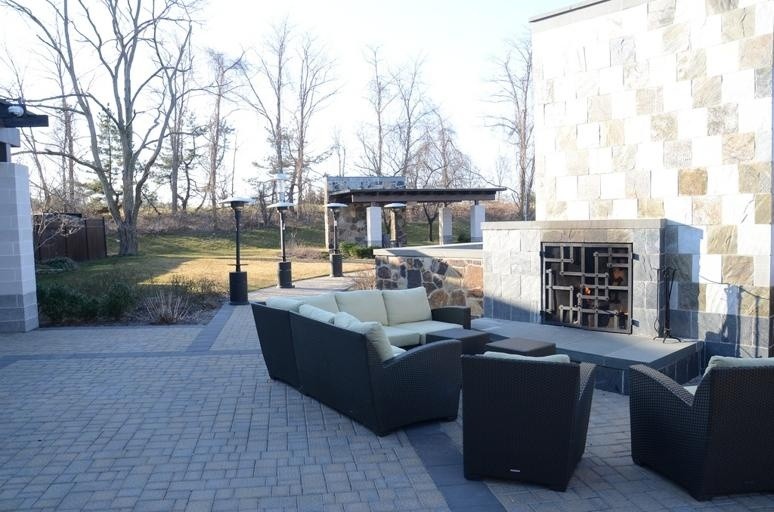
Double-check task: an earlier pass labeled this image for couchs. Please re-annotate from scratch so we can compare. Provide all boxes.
[250,301,471,437]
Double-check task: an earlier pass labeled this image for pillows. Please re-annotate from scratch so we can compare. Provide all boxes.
[265,286,432,362]
[704,355,774,374]
[483,350,571,363]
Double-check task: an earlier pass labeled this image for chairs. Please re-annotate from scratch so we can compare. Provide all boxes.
[628,362,774,503]
[459,353,597,490]
